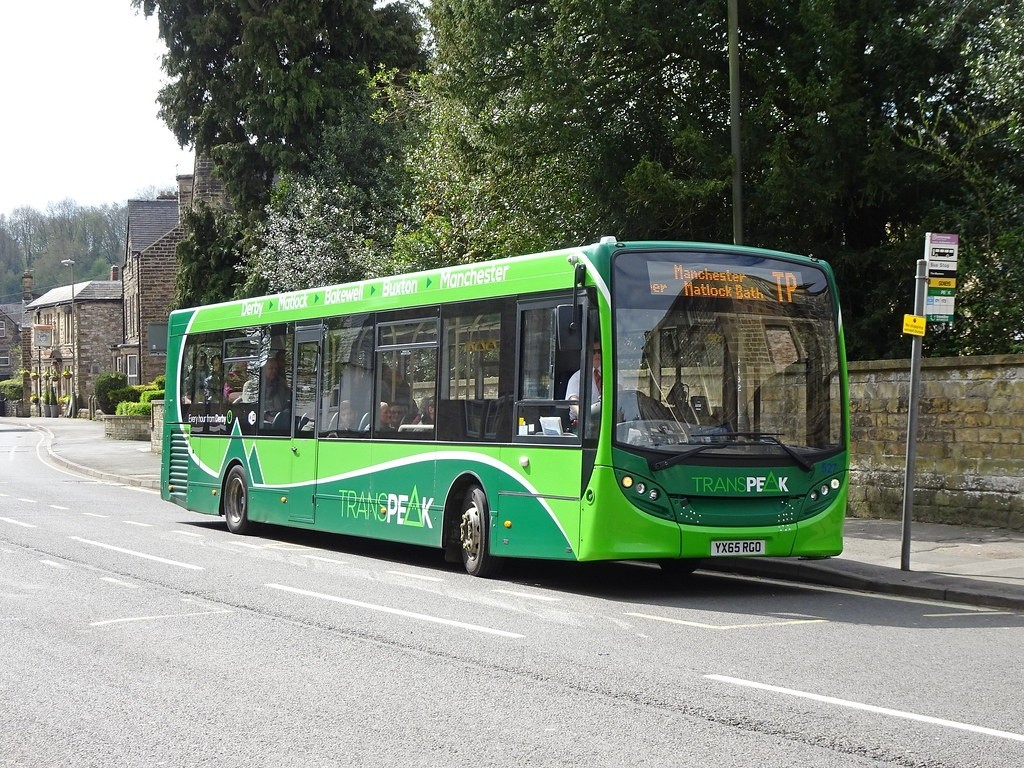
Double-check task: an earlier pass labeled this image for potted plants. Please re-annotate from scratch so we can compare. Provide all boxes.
[49,390,59,417]
[42,391,51,417]
[63,370,72,379]
[20,367,30,376]
[30,371,39,381]
[52,371,59,381]
[43,371,50,380]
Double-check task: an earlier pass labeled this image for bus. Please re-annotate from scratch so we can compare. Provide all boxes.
[161,233,852,579]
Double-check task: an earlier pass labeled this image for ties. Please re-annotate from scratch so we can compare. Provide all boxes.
[594,369,602,395]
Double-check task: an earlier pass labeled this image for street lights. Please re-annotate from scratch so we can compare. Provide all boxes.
[61,259,76,418]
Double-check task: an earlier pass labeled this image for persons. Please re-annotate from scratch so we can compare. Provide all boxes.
[183,351,293,427]
[564,339,623,420]
[337,342,435,431]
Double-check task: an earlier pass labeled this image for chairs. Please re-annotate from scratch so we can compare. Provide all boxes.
[262,409,370,431]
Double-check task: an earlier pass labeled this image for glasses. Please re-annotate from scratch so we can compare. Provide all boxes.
[592,349,602,355]
